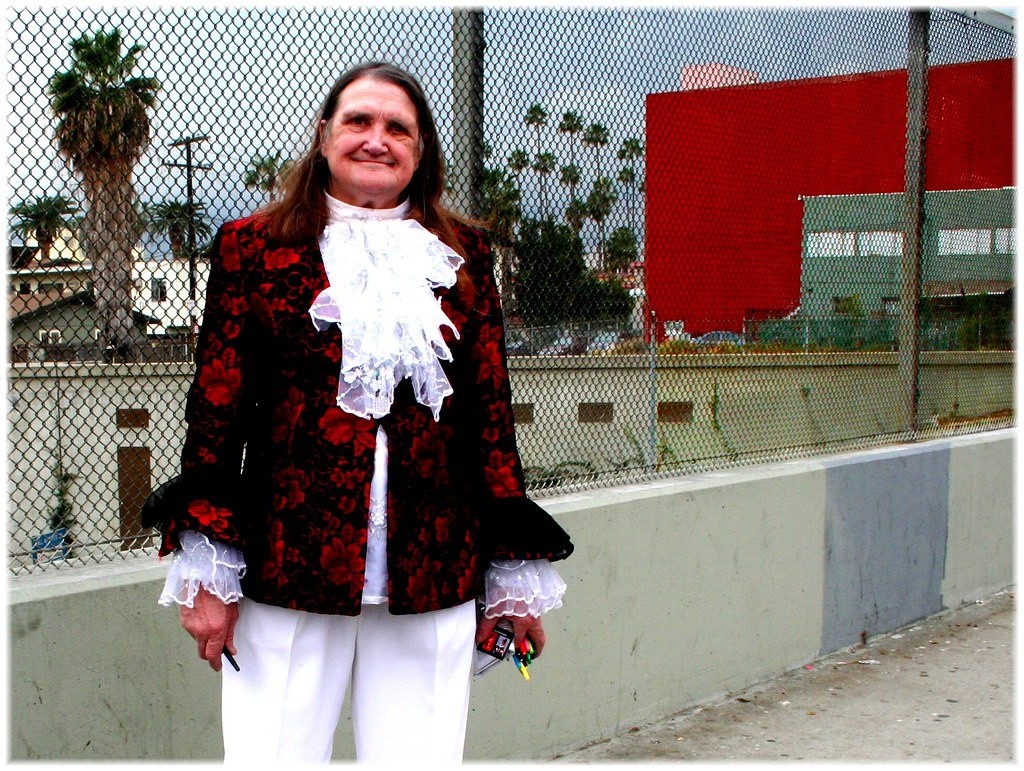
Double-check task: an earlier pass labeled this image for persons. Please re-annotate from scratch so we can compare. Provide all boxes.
[144,60,574,762]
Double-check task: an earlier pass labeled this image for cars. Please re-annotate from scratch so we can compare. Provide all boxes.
[505,339,533,356]
[688,329,747,352]
[536,336,585,356]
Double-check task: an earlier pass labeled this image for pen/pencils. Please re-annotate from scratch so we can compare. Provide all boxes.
[512,635,537,681]
[222,645,241,672]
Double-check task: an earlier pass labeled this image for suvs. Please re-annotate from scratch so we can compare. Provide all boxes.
[584,331,635,356]
